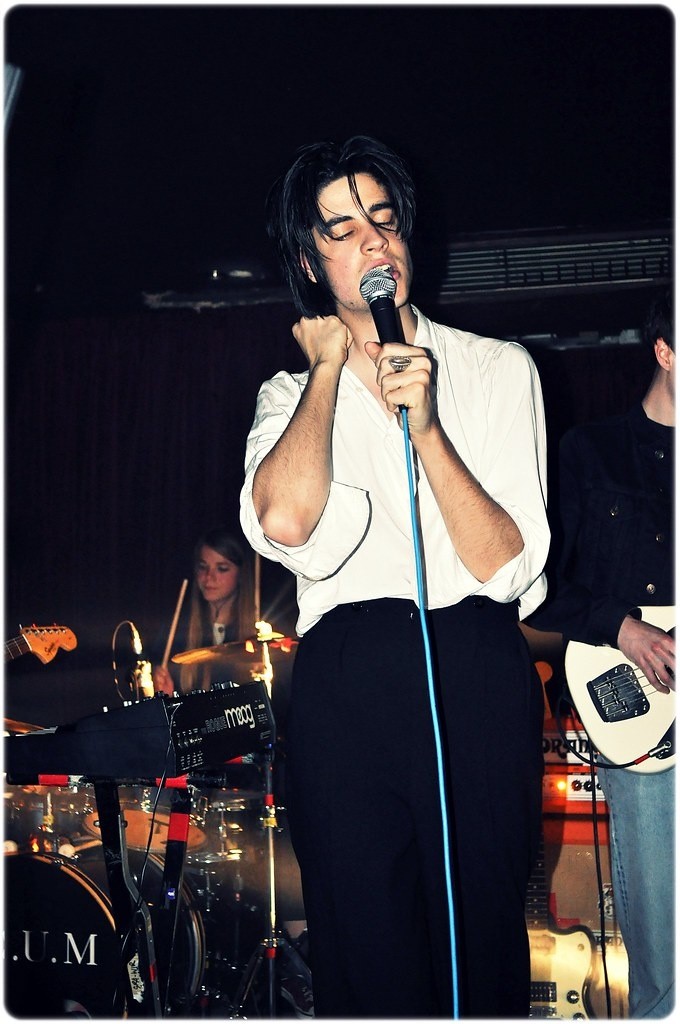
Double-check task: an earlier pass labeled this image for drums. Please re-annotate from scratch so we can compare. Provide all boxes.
[6,715,103,855]
[188,759,268,817]
[81,784,210,855]
[5,834,205,1017]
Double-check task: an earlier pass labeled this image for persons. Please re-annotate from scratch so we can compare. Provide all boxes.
[238,136,550,1019]
[152,531,283,696]
[524,258,676,1019]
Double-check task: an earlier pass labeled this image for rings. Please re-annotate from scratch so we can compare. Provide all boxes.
[389,356,412,373]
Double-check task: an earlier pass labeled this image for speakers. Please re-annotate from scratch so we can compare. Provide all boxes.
[544,816,630,1021]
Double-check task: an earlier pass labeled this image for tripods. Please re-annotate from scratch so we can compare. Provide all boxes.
[231,644,316,1018]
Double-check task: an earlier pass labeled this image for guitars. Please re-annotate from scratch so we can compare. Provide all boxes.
[523,832,596,1019]
[564,607,674,774]
[6,622,78,664]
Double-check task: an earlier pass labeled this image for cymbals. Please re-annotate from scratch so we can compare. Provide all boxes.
[171,636,303,664]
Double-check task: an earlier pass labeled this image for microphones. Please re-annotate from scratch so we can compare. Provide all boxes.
[128,623,154,700]
[359,270,408,414]
